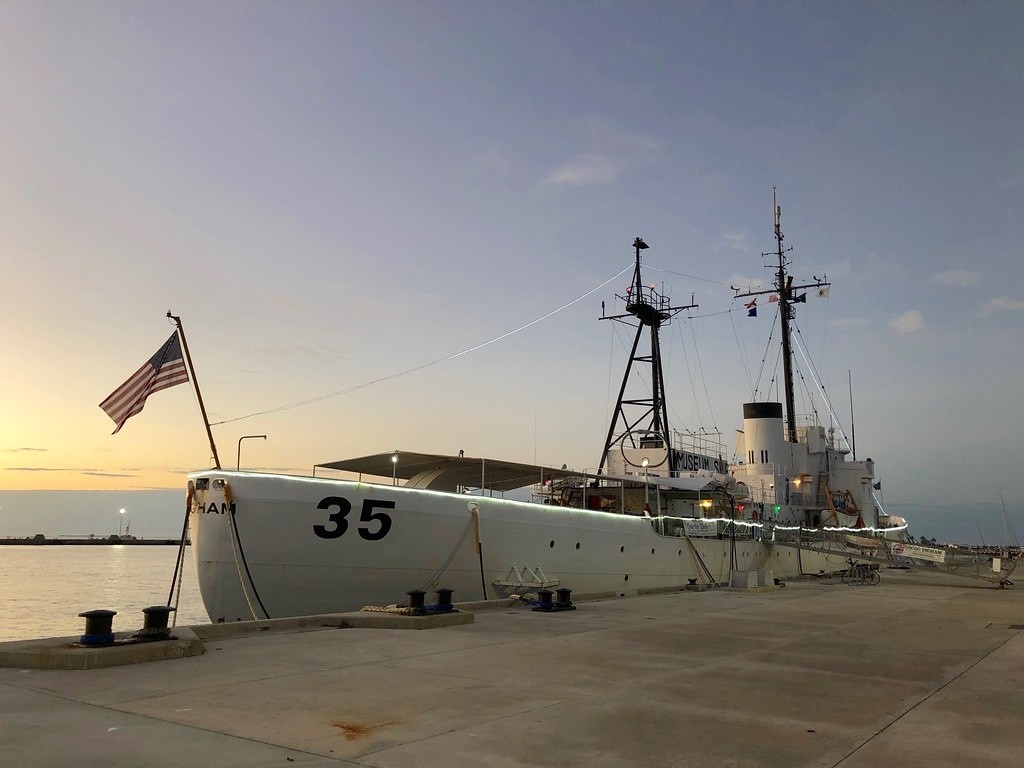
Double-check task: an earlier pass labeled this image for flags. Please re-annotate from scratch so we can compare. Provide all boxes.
[815,286,829,297]
[744,298,757,318]
[796,293,806,303]
[769,295,778,303]
[99,330,189,435]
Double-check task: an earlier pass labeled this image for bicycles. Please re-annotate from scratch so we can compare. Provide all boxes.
[842,557,880,585]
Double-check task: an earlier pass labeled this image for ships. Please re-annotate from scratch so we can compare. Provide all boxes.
[166,185,909,625]
